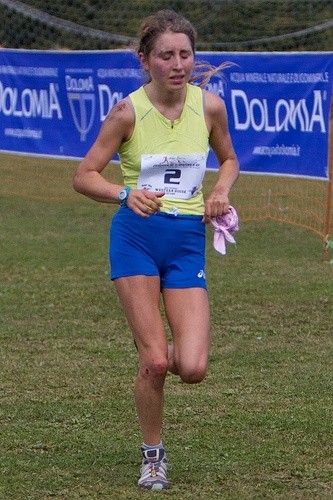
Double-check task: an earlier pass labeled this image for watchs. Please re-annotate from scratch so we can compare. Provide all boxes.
[117,186,131,206]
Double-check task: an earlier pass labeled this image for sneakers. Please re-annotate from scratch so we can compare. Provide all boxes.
[138,446,170,488]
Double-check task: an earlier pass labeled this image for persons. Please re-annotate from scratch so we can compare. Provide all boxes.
[72,8,239,492]
